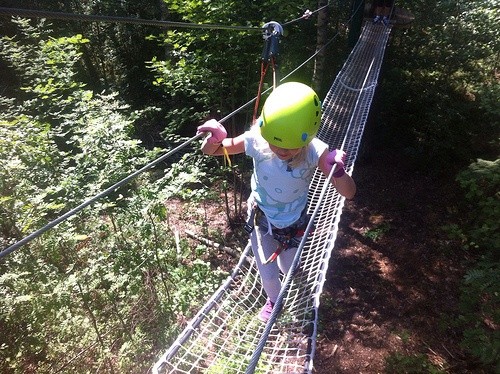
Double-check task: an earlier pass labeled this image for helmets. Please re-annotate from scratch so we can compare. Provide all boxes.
[259,82,322,150]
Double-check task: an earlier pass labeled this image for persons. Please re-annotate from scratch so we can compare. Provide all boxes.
[197,81,356,322]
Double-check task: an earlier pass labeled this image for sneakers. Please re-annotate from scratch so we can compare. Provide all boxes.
[381,19,390,29]
[373,17,381,25]
[258,299,282,323]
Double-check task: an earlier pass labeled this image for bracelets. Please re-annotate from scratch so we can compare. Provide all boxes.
[206,133,232,167]
[332,168,345,178]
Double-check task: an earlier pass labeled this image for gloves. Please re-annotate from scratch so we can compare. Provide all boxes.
[324,150,346,177]
[197,119,227,145]
